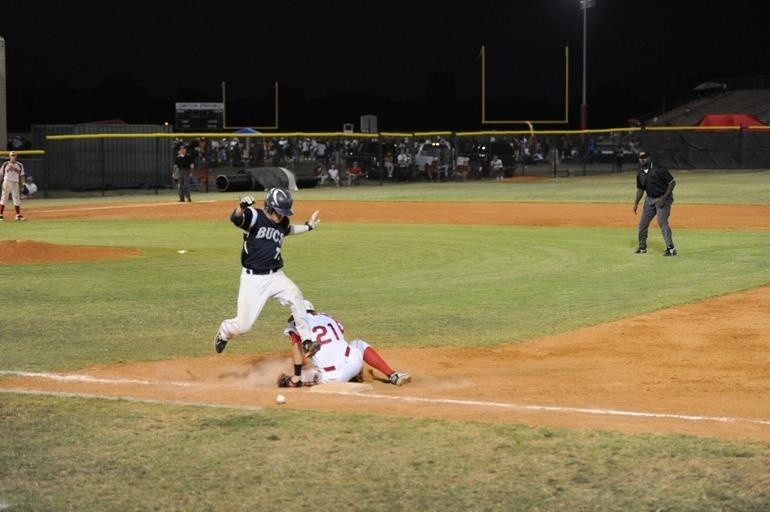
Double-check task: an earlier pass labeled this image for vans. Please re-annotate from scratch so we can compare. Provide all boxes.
[459,137,516,179]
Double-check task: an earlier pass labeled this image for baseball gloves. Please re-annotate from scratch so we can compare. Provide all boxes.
[278,374,302,388]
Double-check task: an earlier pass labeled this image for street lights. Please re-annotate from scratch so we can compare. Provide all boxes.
[580,0,597,142]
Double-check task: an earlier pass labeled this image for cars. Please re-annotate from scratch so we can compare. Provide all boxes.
[592,132,621,162]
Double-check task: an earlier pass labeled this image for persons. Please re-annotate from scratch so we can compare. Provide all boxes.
[275,298,412,389]
[23,174,38,197]
[213,184,322,358]
[0,150,26,221]
[631,151,678,260]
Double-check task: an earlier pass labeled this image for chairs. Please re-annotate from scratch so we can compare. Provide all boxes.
[181,165,246,188]
[297,160,321,186]
[601,150,612,162]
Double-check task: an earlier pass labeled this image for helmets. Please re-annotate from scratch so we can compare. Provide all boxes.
[265,187,296,217]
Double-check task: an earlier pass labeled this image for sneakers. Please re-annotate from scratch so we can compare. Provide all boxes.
[214,319,230,354]
[634,249,649,256]
[303,340,322,359]
[388,370,411,387]
[16,214,27,221]
[0,215,4,220]
[662,247,677,256]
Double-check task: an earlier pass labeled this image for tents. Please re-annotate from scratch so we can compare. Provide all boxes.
[641,81,770,172]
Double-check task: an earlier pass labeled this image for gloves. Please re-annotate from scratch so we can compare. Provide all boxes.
[239,195,256,209]
[309,210,322,231]
[277,372,301,387]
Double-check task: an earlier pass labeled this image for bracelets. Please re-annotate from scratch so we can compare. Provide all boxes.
[304,220,313,231]
[292,363,303,376]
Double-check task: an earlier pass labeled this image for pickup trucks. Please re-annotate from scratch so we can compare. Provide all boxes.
[413,142,452,176]
[345,139,408,182]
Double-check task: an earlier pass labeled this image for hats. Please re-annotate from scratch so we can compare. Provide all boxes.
[9,151,17,156]
[638,151,651,159]
[286,300,317,323]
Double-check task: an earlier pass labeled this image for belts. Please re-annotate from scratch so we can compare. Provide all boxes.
[323,346,351,371]
[246,266,280,275]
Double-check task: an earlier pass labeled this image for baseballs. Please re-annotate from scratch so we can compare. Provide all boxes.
[276,395,285,404]
